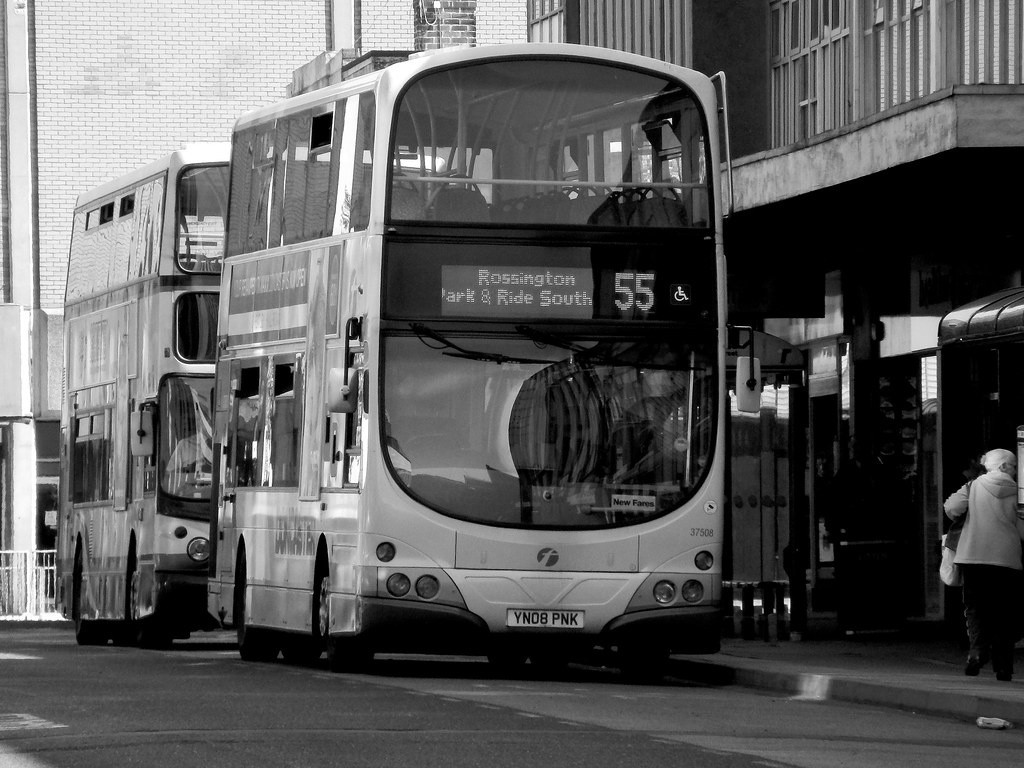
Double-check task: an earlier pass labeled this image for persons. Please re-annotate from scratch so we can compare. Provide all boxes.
[165,413,213,473]
[944,448,1021,682]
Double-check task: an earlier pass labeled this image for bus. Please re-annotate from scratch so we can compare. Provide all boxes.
[207,41,764,679]
[55,143,448,649]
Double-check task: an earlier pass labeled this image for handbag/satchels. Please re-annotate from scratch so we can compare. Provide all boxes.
[940,535,966,587]
[945,480,972,551]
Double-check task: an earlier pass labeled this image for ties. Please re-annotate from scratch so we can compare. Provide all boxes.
[201,437,212,473]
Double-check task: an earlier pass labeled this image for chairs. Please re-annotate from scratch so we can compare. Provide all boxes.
[487,182,689,224]
[361,172,427,223]
[178,254,222,272]
[434,176,493,222]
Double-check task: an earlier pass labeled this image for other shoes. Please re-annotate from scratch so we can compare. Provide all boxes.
[997,674,1012,683]
[966,655,980,678]
